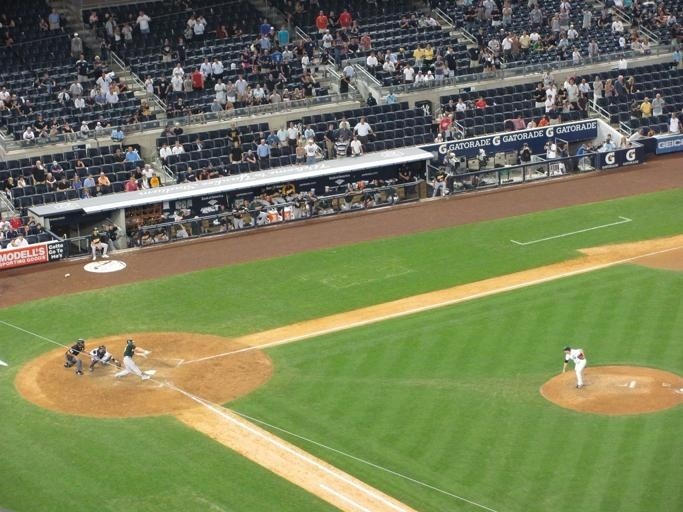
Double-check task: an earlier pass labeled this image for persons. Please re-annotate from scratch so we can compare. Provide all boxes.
[64,339,85,375]
[88,345,114,372]
[114,339,151,380]
[562,346,587,389]
[0,0,682,262]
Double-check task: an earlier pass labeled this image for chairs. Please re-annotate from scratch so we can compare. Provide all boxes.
[0,0,683,250]
